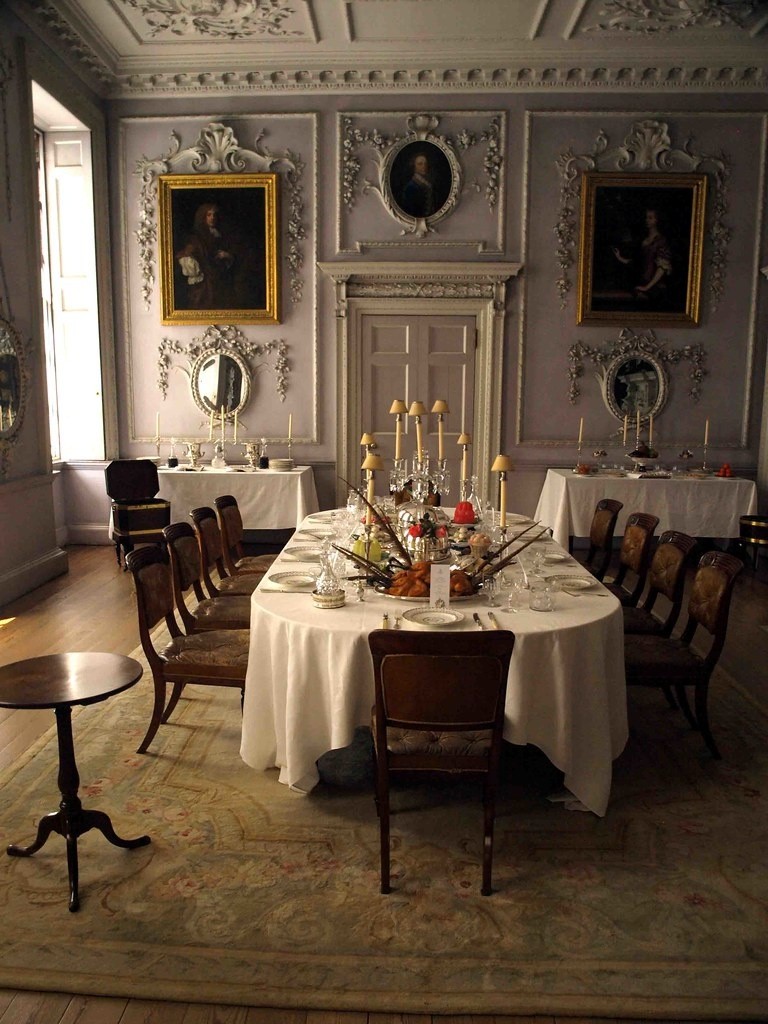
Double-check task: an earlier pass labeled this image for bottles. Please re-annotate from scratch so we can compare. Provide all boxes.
[348,491,367,510]
[356,581,364,602]
[261,438,269,469]
[317,538,339,596]
[468,476,480,515]
[168,439,178,468]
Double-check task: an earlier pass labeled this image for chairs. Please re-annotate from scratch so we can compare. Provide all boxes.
[122,494,737,896]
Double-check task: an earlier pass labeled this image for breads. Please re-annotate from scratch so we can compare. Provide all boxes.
[389,560,474,598]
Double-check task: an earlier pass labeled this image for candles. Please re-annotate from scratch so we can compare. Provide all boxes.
[578,417,584,441]
[704,417,710,445]
[623,411,653,445]
[288,414,293,437]
[209,406,238,442]
[157,411,159,436]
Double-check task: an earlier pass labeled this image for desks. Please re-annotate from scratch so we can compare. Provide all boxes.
[241,508,630,816]
[108,463,319,566]
[534,468,756,557]
[1,651,153,915]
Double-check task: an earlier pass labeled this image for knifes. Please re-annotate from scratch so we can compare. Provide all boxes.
[473,612,500,630]
[279,559,320,563]
[260,589,312,593]
[382,612,388,630]
[294,539,319,542]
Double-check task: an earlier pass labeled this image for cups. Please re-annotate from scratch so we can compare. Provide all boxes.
[374,496,394,509]
[529,583,554,612]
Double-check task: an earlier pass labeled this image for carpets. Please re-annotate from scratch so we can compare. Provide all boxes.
[1,557,768,1022]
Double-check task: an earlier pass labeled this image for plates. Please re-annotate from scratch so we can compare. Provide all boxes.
[270,459,296,472]
[505,515,597,590]
[269,572,317,587]
[402,607,464,625]
[135,458,160,468]
[284,547,322,557]
[298,512,337,536]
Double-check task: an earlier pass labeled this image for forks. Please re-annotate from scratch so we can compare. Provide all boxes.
[562,589,608,597]
[394,610,401,629]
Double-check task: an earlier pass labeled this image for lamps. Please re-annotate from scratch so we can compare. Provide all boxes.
[355,401,512,535]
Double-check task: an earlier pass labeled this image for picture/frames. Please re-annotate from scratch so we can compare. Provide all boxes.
[157,174,282,325]
[378,133,461,229]
[577,171,709,328]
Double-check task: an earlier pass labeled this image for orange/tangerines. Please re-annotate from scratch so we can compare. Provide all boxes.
[719,464,731,477]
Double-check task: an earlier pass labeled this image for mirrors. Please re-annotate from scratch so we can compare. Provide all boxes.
[191,348,253,416]
[0,320,29,438]
[601,351,668,425]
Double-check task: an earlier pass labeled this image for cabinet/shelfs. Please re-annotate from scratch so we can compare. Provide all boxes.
[737,515,768,575]
[104,459,171,571]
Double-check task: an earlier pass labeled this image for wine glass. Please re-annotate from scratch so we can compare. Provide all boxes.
[482,573,521,613]
[530,545,546,575]
